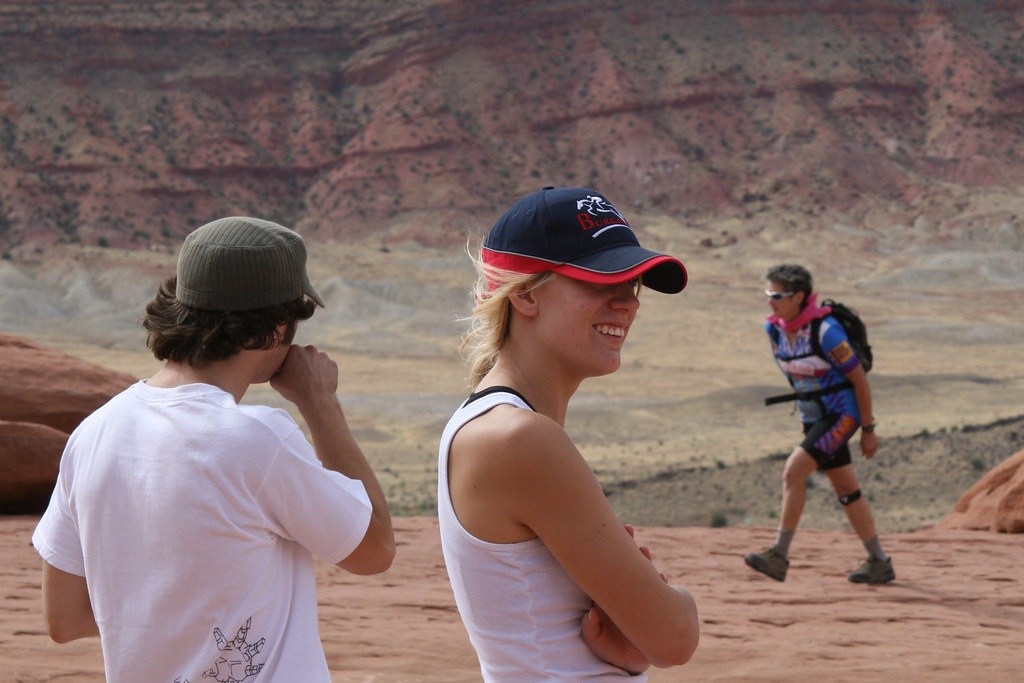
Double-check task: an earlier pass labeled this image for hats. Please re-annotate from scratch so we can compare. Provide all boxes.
[176,215,327,311]
[476,187,688,299]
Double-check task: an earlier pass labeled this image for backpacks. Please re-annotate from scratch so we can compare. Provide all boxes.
[810,299,874,388]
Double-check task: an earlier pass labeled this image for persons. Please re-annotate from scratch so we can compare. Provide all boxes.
[30,215,396,683]
[746,265,895,585]
[440,184,700,683]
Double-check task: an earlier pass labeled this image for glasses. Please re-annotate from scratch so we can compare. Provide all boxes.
[763,288,794,299]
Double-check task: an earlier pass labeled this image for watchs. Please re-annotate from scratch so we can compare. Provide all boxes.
[863,421,876,432]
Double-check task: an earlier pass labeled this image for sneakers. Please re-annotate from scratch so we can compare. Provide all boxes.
[745,548,789,581]
[848,555,895,583]
